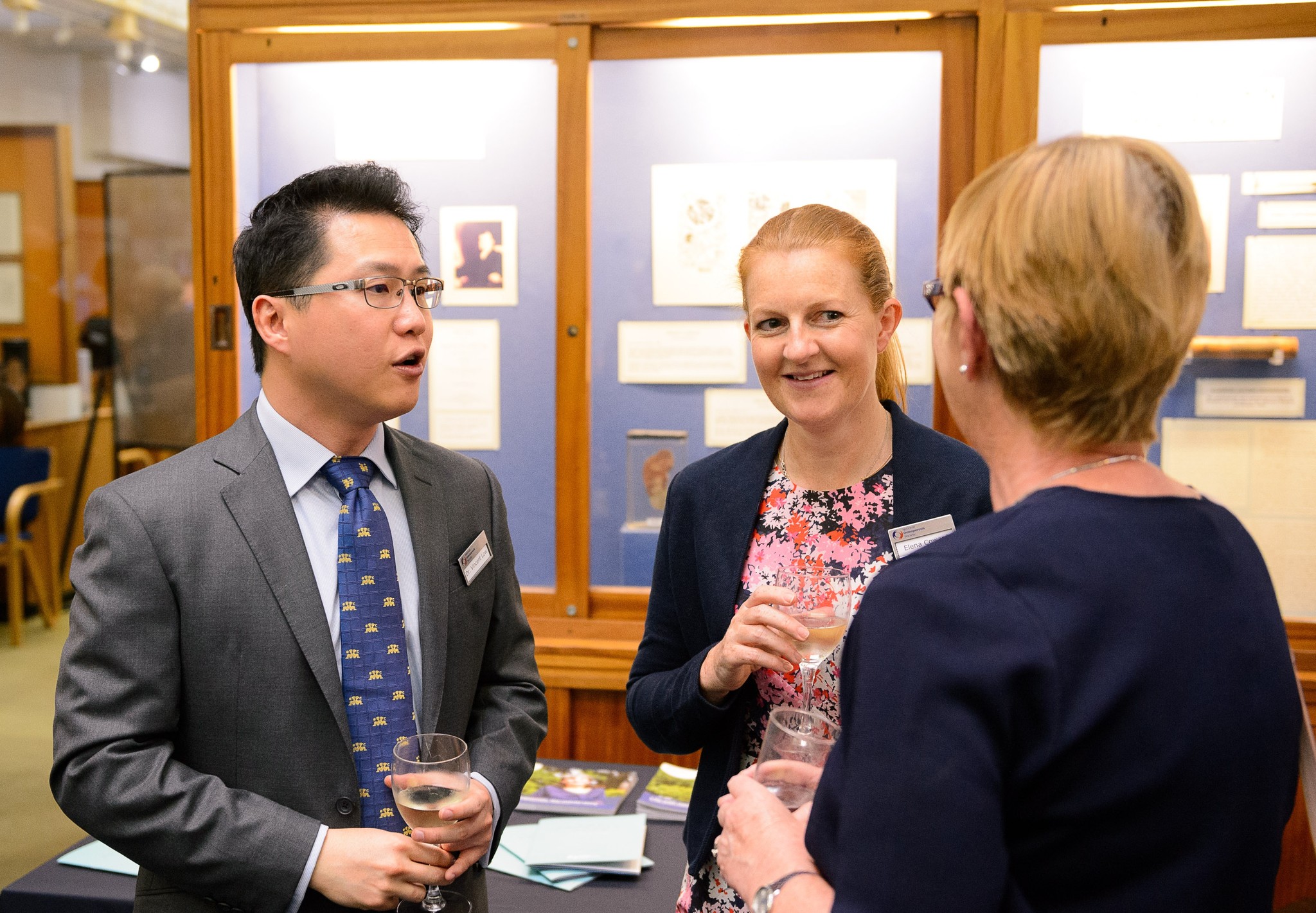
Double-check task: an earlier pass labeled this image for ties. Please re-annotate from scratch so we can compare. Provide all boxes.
[320,455,423,837]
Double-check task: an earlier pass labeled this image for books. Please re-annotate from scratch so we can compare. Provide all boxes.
[514,762,639,816]
[635,762,698,822]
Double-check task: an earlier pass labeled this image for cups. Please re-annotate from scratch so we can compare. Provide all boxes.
[753,706,841,813]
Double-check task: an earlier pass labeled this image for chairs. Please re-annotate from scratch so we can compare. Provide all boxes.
[0,446,64,643]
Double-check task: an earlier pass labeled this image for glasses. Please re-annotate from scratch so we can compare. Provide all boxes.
[922,278,979,313]
[260,276,444,309]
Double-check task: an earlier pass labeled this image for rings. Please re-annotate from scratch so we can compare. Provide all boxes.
[712,838,722,857]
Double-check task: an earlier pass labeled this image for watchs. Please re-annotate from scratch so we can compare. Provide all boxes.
[752,871,821,913]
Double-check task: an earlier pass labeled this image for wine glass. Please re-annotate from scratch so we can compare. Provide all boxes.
[392,733,474,913]
[773,565,851,755]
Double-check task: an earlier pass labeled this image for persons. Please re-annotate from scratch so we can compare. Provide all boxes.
[624,203,996,913]
[50,164,547,913]
[714,136,1304,912]
[455,231,502,289]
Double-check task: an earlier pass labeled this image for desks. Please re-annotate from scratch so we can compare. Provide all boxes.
[0,760,700,913]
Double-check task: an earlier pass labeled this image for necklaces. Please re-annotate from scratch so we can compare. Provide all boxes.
[778,410,892,482]
[1039,455,1148,486]
